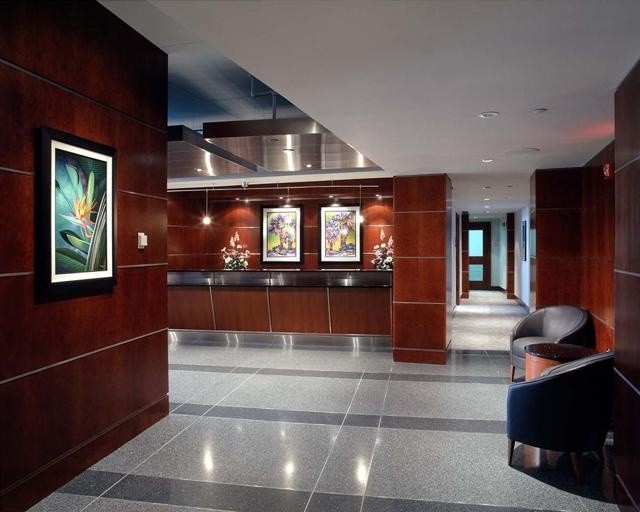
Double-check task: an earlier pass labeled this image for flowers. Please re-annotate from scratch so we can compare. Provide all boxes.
[370,229,394,269]
[221,232,249,269]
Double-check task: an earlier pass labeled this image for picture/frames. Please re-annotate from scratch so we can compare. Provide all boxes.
[260,204,304,264]
[35,127,117,305]
[319,203,363,264]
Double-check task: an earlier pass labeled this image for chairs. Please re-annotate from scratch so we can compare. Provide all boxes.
[510,305,588,383]
[507,352,615,486]
